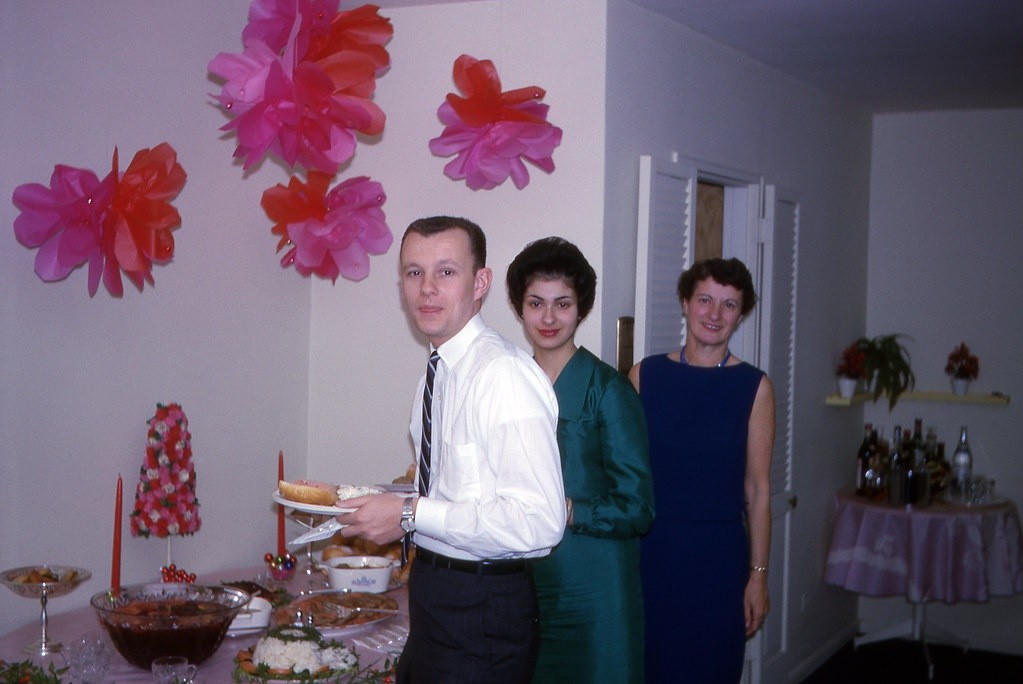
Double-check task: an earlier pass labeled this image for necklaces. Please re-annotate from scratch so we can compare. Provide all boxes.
[679,346,731,368]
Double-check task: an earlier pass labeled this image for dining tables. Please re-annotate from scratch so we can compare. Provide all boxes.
[0,564,411,684]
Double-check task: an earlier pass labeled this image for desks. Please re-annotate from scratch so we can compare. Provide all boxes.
[825,493,1023,680]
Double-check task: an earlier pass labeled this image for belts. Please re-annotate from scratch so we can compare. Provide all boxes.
[414,544,533,574]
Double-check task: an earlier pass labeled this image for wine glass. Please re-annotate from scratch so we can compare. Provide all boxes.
[0,563,93,656]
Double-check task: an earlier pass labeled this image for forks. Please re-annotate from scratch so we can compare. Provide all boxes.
[323,601,401,620]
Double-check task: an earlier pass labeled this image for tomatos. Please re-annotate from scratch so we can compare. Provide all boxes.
[238,649,329,675]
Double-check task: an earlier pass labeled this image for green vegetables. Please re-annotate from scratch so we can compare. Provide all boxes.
[254,624,359,681]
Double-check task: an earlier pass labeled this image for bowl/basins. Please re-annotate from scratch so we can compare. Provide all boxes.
[318,556,401,593]
[226,596,272,638]
[89,582,252,670]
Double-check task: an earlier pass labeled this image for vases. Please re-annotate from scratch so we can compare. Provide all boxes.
[838,379,855,398]
[952,379,968,396]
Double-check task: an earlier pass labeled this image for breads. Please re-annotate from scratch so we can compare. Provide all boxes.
[393,463,417,484]
[278,480,340,506]
[322,529,414,585]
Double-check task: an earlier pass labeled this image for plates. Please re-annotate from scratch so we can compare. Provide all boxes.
[272,494,358,516]
[287,590,399,639]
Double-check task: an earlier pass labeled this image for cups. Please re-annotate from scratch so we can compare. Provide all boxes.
[151,657,198,684]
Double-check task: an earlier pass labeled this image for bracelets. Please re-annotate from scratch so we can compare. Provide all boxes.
[750,566,768,572]
[566,498,572,524]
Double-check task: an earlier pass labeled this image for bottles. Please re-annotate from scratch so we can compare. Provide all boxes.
[854,422,972,506]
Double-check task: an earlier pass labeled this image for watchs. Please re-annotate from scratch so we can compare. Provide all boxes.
[400,497,416,533]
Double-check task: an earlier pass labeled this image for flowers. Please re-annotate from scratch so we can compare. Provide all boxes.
[11,140,187,300]
[943,340,980,380]
[836,331,915,414]
[129,400,203,539]
[204,0,394,285]
[429,50,563,192]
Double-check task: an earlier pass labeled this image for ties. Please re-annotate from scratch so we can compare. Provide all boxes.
[400,349,442,570]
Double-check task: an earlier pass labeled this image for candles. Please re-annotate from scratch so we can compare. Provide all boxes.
[110,472,125,595]
[277,449,286,556]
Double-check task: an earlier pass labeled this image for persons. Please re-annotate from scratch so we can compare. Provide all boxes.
[506,236,652,684]
[334,217,568,684]
[627,258,775,684]
